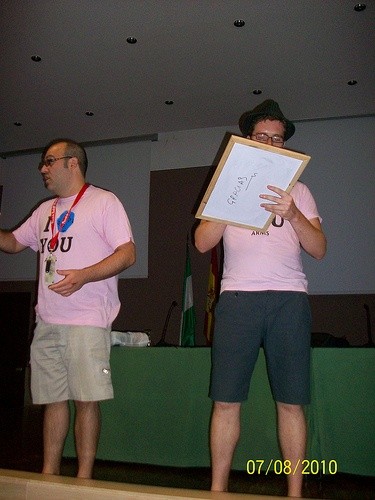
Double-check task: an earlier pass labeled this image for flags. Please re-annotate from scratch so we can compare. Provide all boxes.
[178,246,196,348]
[203,246,219,347]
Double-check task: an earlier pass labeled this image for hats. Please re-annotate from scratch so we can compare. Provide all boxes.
[239,99,295,142]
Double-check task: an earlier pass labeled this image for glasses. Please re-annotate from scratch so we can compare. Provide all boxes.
[252,132,285,144]
[37,156,72,170]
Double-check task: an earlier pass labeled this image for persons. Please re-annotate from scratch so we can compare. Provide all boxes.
[195,99,327,498]
[0,139,136,479]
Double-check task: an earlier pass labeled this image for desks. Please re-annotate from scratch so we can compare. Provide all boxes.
[61,346,375,498]
